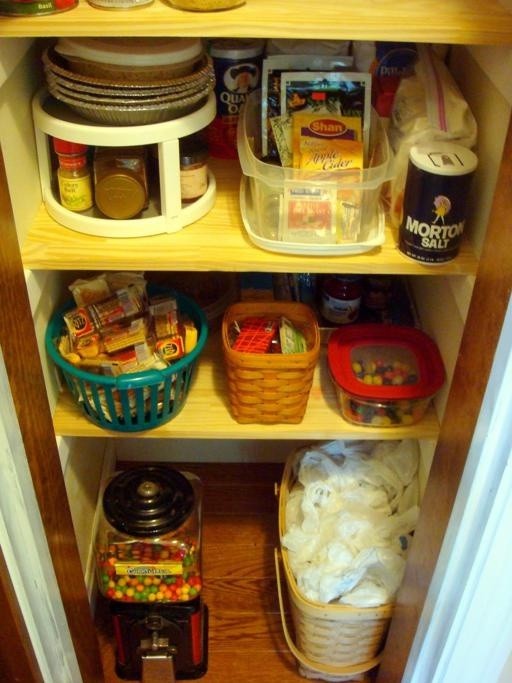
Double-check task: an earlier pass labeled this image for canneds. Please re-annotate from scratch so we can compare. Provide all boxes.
[180,139,210,201]
[210,48,267,163]
[319,275,363,327]
[89,150,152,222]
[395,141,478,268]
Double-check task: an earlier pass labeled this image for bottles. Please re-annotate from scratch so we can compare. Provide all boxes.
[54,138,209,219]
[88,467,203,602]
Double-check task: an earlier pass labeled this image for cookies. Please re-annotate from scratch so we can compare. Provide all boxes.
[62,272,202,424]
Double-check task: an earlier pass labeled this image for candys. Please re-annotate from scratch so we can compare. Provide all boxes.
[344,358,428,427]
[93,534,202,604]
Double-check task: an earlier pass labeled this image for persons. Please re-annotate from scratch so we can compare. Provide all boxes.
[287,89,309,114]
[229,63,256,96]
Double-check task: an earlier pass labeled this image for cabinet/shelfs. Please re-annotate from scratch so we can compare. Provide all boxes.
[1,0,512,683]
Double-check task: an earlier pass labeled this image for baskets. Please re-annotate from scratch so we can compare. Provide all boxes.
[273,444,398,683]
[220,302,322,424]
[46,281,210,434]
